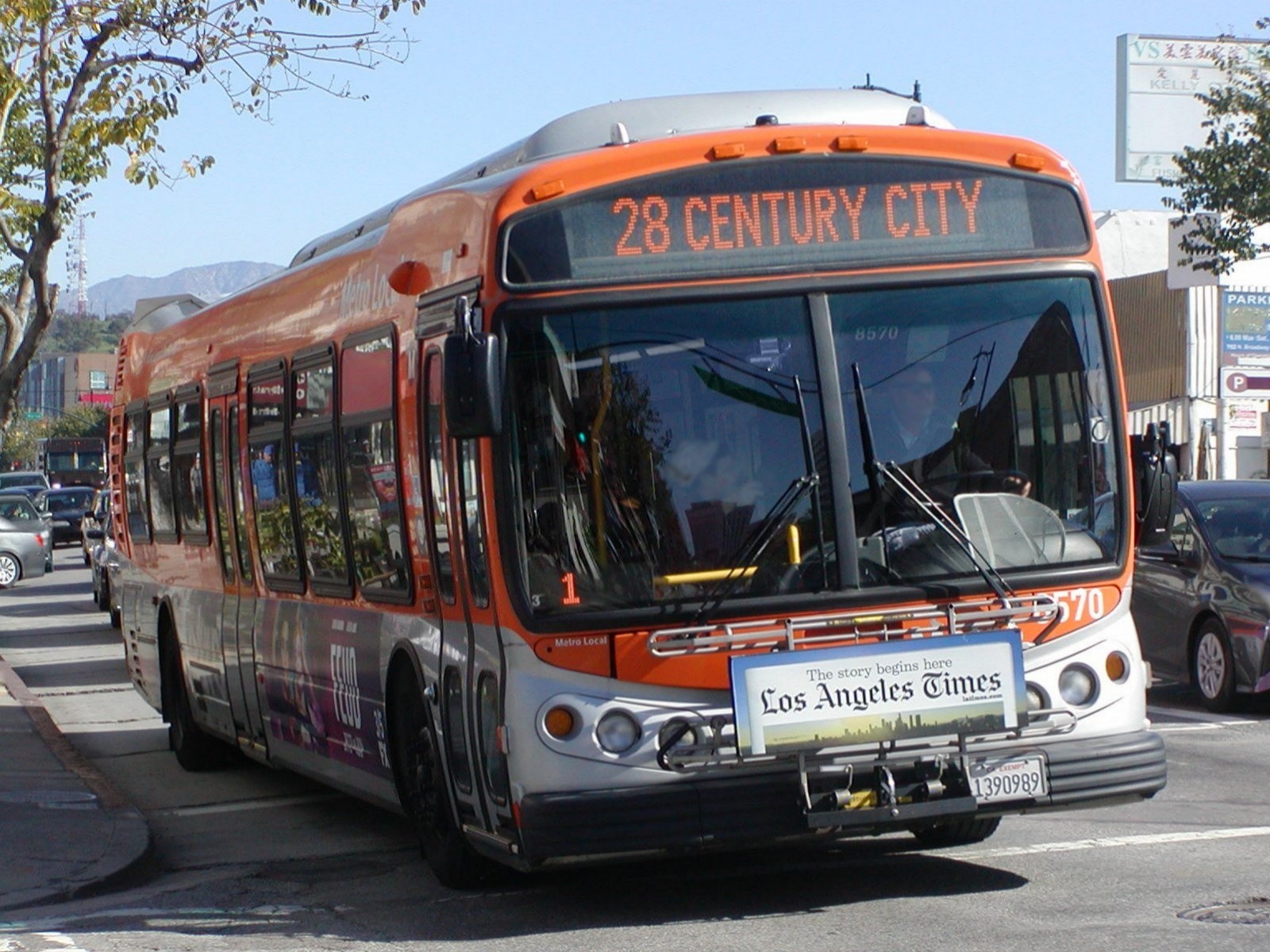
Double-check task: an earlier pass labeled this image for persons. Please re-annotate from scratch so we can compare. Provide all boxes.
[878,366,1032,500]
[251,443,318,501]
[191,458,203,513]
[661,438,764,562]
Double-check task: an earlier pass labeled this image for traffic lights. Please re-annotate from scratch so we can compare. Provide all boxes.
[14,460,19,468]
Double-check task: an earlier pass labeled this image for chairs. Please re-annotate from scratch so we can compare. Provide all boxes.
[1212,507,1264,553]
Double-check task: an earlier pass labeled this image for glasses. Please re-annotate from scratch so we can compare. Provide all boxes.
[895,381,936,395]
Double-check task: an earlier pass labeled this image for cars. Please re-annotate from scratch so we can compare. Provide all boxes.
[1125,475,1269,716]
[84,504,126,627]
[0,472,114,589]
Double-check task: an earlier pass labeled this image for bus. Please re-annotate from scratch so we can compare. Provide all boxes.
[105,74,1173,895]
[45,434,103,494]
